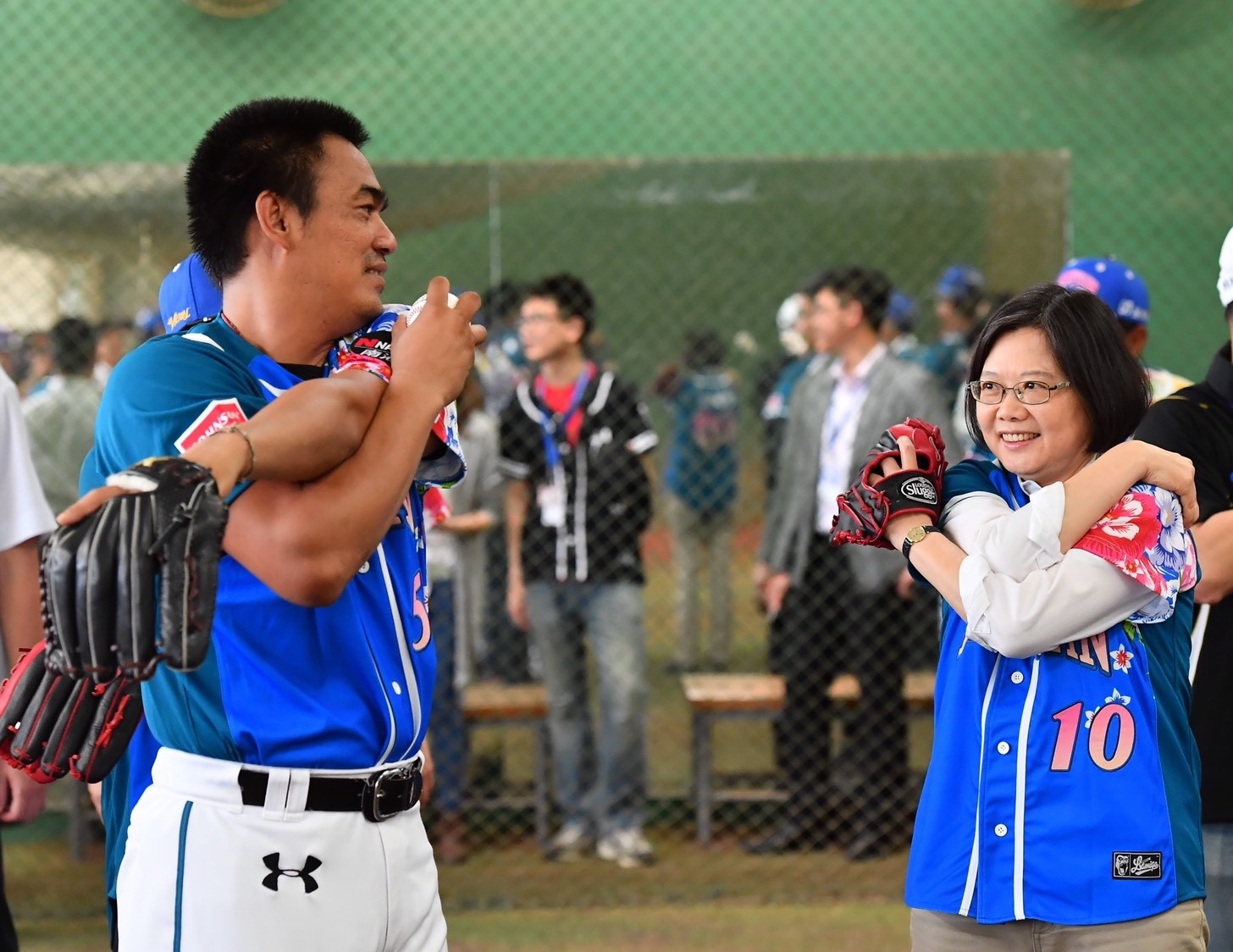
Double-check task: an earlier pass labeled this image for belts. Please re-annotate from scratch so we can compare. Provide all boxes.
[238,757,425,823]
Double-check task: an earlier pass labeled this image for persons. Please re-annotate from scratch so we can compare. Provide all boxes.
[0,229,1232,952]
[53,98,488,951]
[499,276,664,869]
[865,282,1212,952]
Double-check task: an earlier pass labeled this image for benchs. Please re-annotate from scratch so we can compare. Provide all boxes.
[680,668,937,851]
[451,680,553,846]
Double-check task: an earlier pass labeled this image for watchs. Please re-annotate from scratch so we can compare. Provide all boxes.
[901,524,943,561]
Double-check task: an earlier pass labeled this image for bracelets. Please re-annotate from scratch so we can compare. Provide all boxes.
[210,422,256,485]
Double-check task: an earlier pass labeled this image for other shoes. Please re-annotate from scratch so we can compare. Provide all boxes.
[737,826,826,857]
[600,823,653,867]
[846,825,892,860]
[543,818,597,859]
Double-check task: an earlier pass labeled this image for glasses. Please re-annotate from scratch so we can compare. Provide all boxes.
[964,375,1073,406]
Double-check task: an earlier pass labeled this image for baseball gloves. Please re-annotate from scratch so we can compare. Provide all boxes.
[2,638,144,785]
[38,455,232,684]
[826,415,948,549]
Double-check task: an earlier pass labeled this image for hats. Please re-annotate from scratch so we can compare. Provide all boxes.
[933,269,987,313]
[1056,257,1150,325]
[886,295,917,332]
